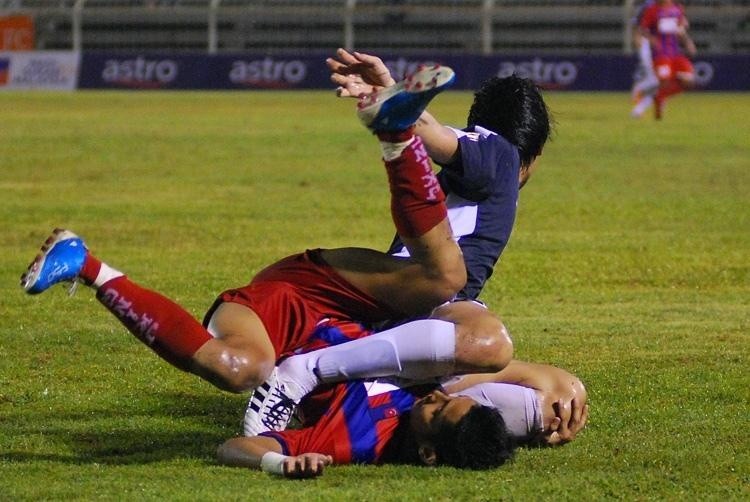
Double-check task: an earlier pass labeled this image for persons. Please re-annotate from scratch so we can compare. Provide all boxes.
[19,60,590,481]
[638,1,700,119]
[322,38,591,447]
[628,1,661,120]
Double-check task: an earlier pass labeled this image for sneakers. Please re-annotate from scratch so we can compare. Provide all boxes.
[356,65,456,134]
[22,227,90,295]
[244,367,298,436]
